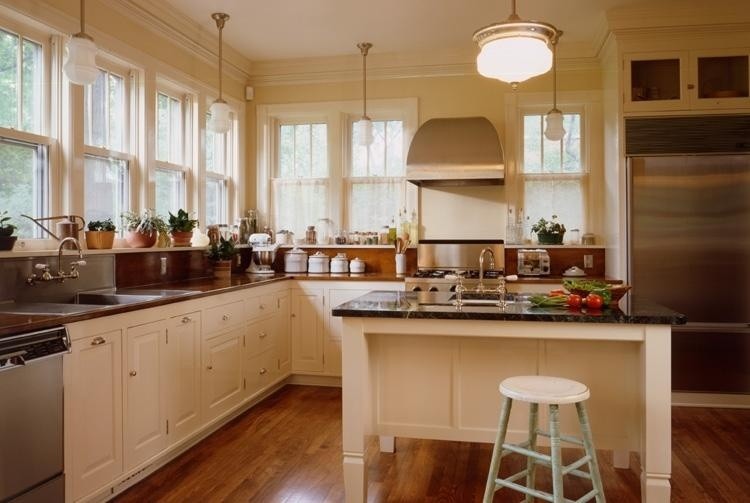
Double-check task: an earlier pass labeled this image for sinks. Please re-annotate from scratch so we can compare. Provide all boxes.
[12,291,162,310]
[449,288,520,304]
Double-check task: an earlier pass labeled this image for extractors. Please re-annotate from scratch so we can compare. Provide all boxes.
[408,119,504,188]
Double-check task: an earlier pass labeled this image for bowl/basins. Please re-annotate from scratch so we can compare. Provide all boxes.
[567,283,632,305]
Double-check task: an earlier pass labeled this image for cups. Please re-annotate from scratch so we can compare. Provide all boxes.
[285,246,308,272]
[331,252,349,273]
[309,251,329,272]
[350,256,366,272]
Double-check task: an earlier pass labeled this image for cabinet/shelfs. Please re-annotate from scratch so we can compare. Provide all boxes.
[200,276,287,440]
[165,296,205,464]
[620,45,750,114]
[286,275,324,386]
[63,312,124,503]
[123,302,166,491]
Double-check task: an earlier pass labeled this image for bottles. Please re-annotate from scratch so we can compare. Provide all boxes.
[509,207,533,245]
[207,222,239,244]
[305,217,418,245]
[572,228,579,244]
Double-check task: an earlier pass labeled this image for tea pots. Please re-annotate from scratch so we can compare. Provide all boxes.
[249,234,278,272]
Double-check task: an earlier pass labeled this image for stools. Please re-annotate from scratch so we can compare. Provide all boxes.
[481,375,606,503]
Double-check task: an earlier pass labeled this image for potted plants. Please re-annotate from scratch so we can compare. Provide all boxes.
[1,209,19,250]
[530,214,566,244]
[207,233,242,279]
[83,206,199,248]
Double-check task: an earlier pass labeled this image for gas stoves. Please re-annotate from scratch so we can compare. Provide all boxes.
[408,267,501,281]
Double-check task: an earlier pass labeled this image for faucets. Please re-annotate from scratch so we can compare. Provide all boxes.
[58,237,83,278]
[477,248,495,289]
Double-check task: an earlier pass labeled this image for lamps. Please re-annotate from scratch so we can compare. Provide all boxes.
[207,10,235,133]
[472,0,567,142]
[64,0,99,84]
[355,41,375,145]
